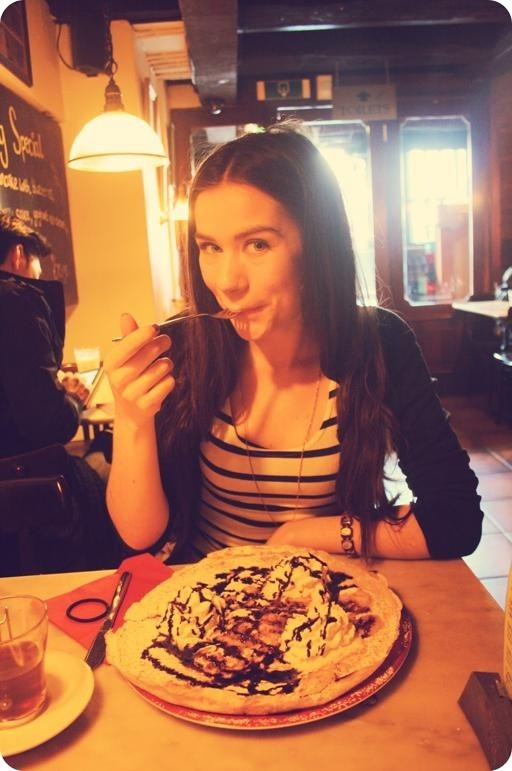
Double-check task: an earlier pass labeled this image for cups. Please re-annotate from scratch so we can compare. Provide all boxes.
[0,593,50,727]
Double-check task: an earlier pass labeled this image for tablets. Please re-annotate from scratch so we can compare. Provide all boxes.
[79,368,104,412]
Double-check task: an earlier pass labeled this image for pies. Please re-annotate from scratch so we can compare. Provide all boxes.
[103,544,404,716]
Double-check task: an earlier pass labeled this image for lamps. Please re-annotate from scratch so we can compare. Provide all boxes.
[69,60,170,173]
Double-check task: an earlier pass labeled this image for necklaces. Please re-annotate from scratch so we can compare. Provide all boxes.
[238,352,325,526]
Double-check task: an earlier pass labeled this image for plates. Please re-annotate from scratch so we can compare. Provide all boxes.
[0,647,96,758]
[125,584,416,732]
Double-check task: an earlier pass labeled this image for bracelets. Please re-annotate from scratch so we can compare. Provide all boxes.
[337,511,361,563]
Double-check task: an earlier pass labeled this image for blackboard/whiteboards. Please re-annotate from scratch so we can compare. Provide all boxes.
[0,84,78,303]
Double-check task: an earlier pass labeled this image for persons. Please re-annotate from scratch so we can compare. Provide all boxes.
[0,210,113,539]
[102,119,487,568]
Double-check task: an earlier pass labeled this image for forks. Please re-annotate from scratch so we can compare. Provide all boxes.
[108,306,244,347]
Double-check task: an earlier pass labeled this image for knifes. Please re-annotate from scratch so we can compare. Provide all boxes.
[84,571,132,670]
